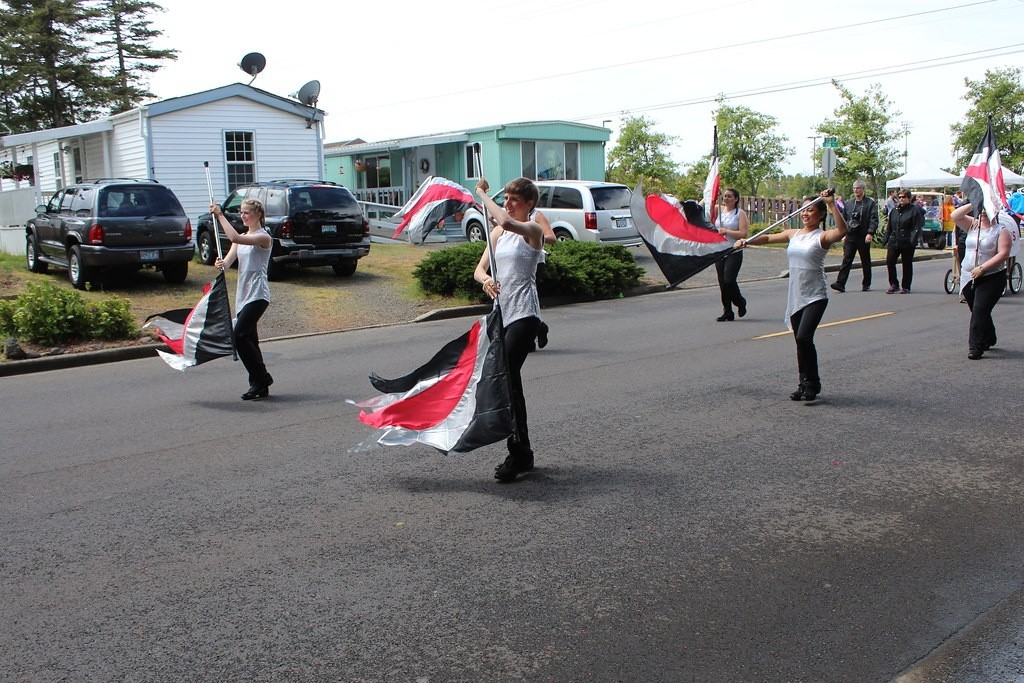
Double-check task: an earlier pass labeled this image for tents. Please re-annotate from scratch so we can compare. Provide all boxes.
[886,165,1024,199]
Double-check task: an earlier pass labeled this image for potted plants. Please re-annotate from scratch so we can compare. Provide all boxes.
[0,160,34,181]
[354,159,365,172]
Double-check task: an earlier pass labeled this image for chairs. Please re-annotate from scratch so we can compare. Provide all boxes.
[300,197,310,208]
[120,201,133,210]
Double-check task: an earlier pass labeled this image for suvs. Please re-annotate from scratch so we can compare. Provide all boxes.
[25,177,196,290]
[460,178,644,251]
[197,179,372,281]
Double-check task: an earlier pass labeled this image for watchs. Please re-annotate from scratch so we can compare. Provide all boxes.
[979,265,985,275]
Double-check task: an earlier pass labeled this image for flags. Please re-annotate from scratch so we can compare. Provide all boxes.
[703,125,722,225]
[143,268,236,372]
[346,304,518,454]
[392,175,477,245]
[961,121,1024,222]
[628,181,733,289]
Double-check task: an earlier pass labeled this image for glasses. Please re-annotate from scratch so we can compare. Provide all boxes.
[898,195,907,199]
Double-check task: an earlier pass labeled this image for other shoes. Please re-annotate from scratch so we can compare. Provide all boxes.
[264,373,273,386]
[900,289,911,294]
[494,453,535,480]
[860,285,871,291]
[789,383,822,400]
[968,339,996,359]
[716,310,734,322]
[239,383,274,400]
[886,285,900,294]
[738,298,746,317]
[830,282,845,292]
[528,322,549,352]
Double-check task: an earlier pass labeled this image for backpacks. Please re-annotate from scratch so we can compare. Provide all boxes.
[883,198,892,215]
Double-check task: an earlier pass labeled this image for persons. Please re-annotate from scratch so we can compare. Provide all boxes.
[697,187,748,322]
[831,180,1024,295]
[475,177,556,481]
[949,202,1012,359]
[216,197,274,401]
[735,190,847,400]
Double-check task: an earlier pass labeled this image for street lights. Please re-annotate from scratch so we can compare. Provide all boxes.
[601,119,613,128]
[901,121,914,174]
[808,136,822,176]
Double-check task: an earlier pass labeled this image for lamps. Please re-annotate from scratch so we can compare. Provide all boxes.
[20,146,25,152]
